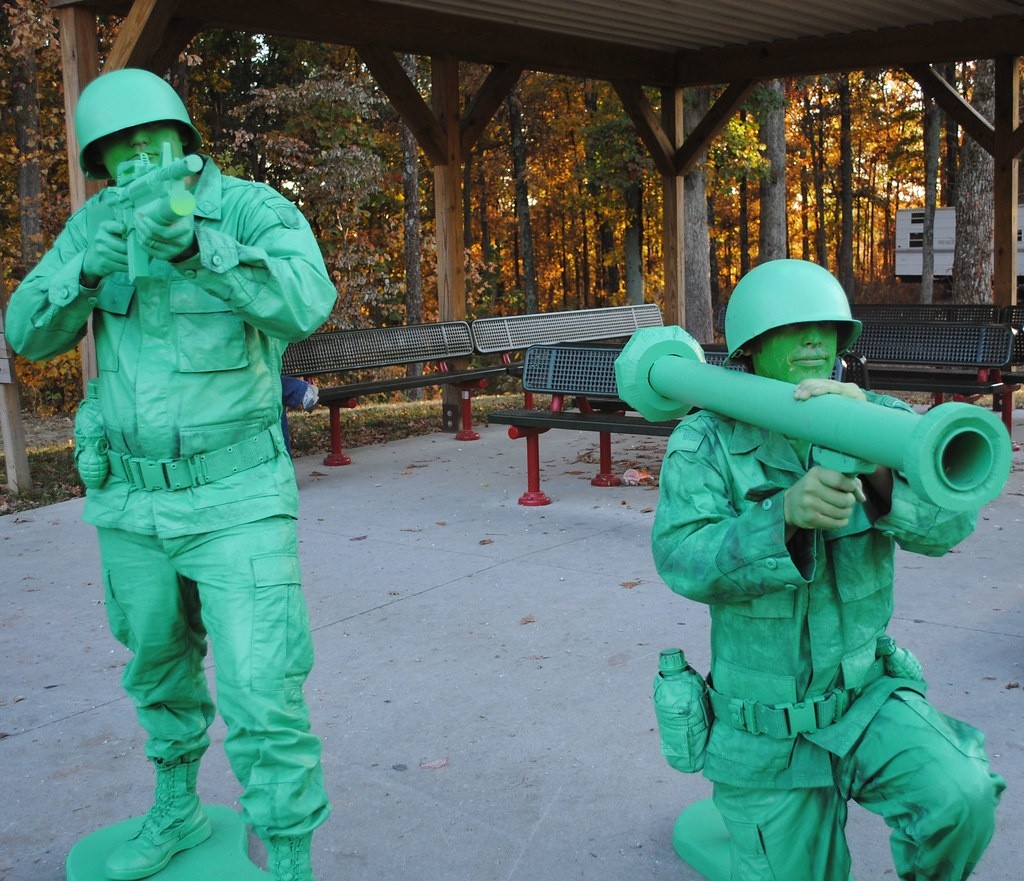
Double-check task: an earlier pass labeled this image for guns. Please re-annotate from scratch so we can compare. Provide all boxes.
[105,142,204,287]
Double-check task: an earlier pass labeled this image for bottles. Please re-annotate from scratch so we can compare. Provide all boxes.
[654,647,709,774]
[75,377,104,435]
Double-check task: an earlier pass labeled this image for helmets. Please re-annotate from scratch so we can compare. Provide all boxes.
[75,68,202,181]
[726,260,862,367]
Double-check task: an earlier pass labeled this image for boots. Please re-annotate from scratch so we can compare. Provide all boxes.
[107,760,214,880]
[268,831,316,881]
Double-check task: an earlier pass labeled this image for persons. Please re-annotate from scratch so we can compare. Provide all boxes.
[6,69,337,881]
[280,376,319,461]
[652,259,1006,881]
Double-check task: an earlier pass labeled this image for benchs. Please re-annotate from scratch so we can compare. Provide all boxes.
[850,303,1024,451]
[471,304,664,379]
[279,321,523,466]
[486,344,847,507]
